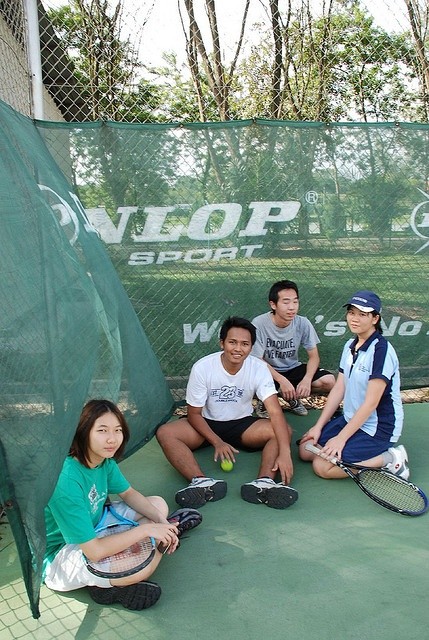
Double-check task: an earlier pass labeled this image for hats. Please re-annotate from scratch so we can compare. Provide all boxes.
[342,290,383,313]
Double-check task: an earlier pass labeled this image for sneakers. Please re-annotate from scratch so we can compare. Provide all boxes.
[166,508,203,550]
[257,400,269,418]
[289,399,308,415]
[381,445,410,480]
[89,581,162,612]
[175,476,227,509]
[241,478,299,511]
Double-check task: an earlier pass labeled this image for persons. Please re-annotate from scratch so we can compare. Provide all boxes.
[295,288,410,482]
[250,279,336,419]
[28,399,202,611]
[155,314,300,509]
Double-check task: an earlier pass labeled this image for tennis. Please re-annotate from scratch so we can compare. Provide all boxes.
[221,458,232,472]
